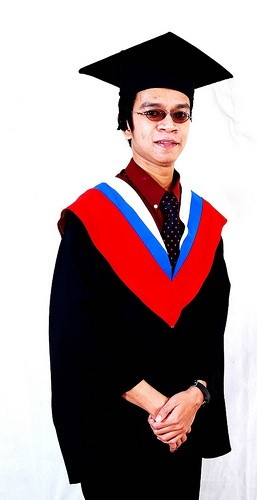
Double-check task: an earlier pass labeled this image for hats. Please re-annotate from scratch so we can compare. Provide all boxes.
[79,32,234,100]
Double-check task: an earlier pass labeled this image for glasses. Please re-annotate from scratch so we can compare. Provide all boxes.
[131,109,192,123]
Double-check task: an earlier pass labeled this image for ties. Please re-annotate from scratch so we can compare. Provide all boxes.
[160,192,185,279]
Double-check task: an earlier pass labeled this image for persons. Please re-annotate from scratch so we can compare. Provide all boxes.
[49,31,232,500]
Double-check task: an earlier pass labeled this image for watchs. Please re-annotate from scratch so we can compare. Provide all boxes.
[189,379,212,406]
